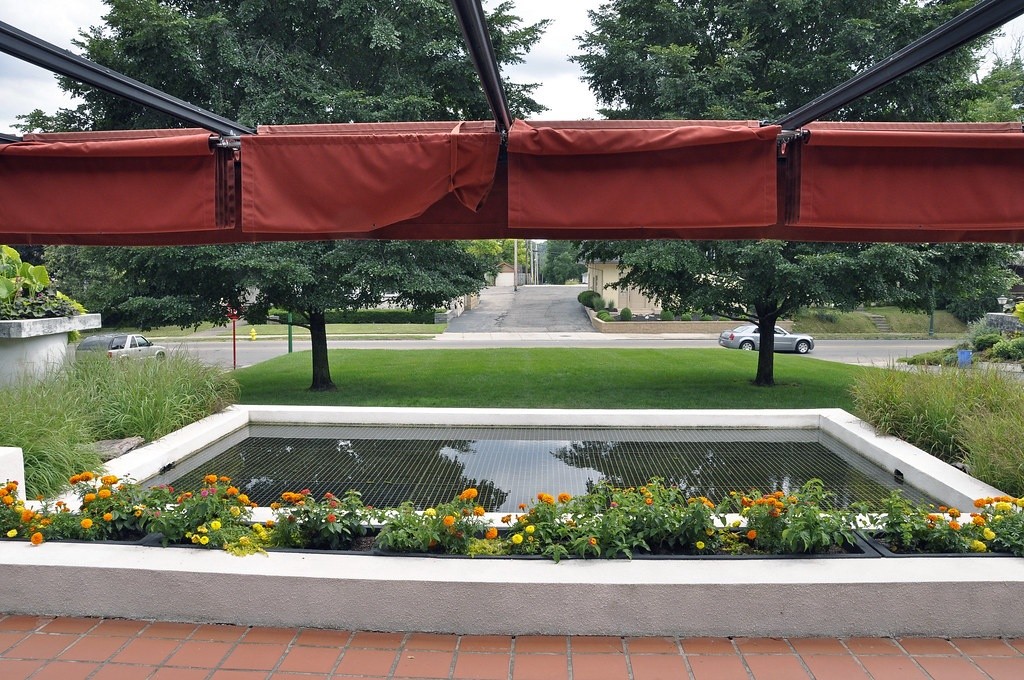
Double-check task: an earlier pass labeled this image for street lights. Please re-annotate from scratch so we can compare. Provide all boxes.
[997,295,1008,336]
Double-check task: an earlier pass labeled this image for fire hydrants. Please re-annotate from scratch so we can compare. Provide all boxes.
[250,328,257,340]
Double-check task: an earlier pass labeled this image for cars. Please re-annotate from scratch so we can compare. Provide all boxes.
[719,319,816,354]
[75,334,167,366]
